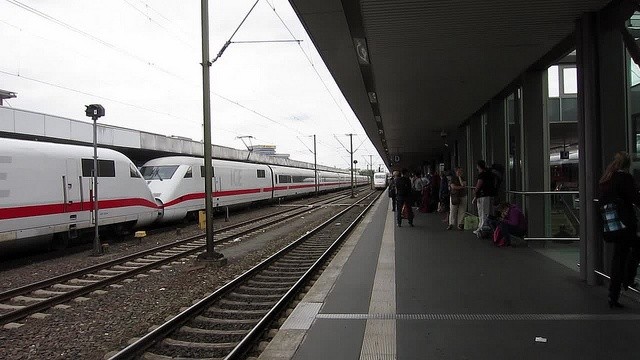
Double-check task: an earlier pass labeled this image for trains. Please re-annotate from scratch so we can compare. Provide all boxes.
[374,173,387,187]
[1,138,160,245]
[139,156,372,221]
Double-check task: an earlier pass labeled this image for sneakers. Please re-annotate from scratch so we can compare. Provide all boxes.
[445,225,452,230]
[457,225,462,230]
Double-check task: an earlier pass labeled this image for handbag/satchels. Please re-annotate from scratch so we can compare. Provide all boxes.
[592,199,640,244]
[401,202,409,219]
[437,199,449,212]
[494,227,511,246]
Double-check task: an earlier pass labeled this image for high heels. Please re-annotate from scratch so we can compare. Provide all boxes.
[624,278,635,289]
[608,297,623,309]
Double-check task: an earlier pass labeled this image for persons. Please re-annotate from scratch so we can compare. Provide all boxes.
[472,160,490,239]
[446,166,467,230]
[493,200,526,247]
[598,150,640,310]
[395,168,414,227]
[411,168,439,214]
[389,171,401,212]
